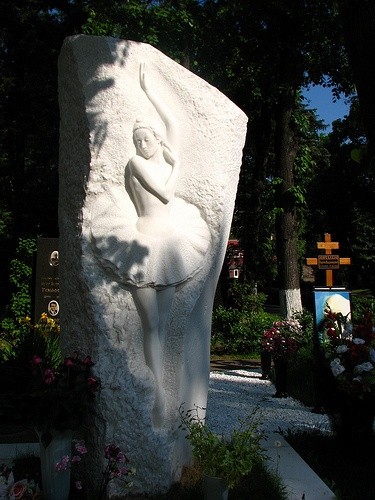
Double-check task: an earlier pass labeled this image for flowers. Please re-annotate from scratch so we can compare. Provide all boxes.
[176,412,270,487]
[260,305,375,391]
[51,439,139,500]
[19,351,102,444]
[8,477,38,499]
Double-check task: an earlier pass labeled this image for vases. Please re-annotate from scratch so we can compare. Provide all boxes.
[202,473,233,500]
[260,350,272,380]
[36,430,74,500]
[272,354,290,399]
[322,381,375,464]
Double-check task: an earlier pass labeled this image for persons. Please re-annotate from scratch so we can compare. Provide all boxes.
[91,61,213,427]
[49,302,57,315]
